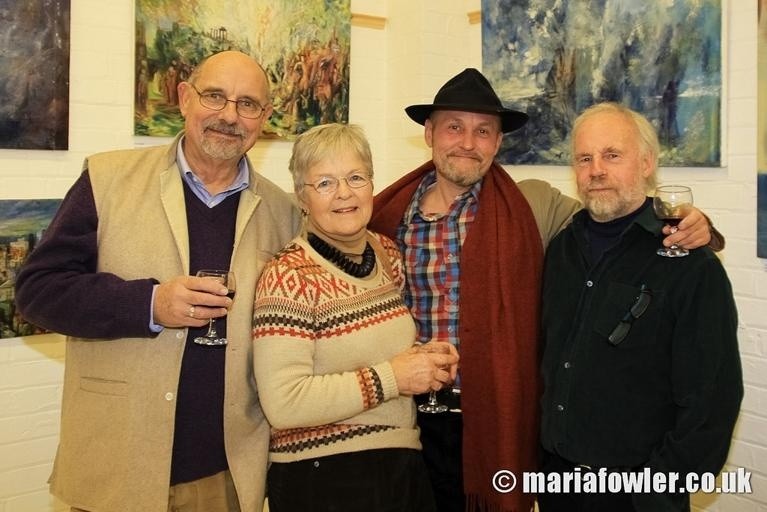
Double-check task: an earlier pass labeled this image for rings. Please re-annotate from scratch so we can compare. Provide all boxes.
[188,305,195,318]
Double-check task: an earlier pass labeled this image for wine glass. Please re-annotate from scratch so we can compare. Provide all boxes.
[654,184,692,259]
[409,344,452,417]
[189,269,235,346]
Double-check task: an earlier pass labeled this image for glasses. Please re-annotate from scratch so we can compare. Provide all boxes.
[305,174,373,196]
[608,290,651,347]
[190,82,265,119]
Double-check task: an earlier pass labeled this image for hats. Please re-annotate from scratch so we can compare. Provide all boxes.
[405,68,530,134]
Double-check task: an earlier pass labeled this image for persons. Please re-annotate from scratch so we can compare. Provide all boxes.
[11,49,306,509]
[536,100,746,511]
[363,67,727,510]
[250,121,460,511]
